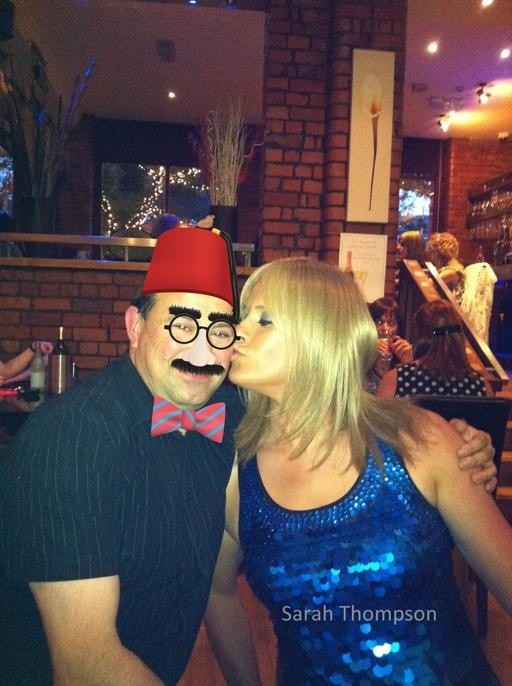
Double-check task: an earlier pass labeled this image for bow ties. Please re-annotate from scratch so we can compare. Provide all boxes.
[151,396,225,444]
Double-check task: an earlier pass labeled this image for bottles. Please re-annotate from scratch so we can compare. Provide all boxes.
[30,340,46,392]
[344,251,354,279]
[48,326,71,398]
[470,189,512,266]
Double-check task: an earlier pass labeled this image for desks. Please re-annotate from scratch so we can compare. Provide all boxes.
[0,386,59,418]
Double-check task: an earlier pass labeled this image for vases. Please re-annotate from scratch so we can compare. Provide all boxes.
[21,197,54,257]
[208,206,237,242]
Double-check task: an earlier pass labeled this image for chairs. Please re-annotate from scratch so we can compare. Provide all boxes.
[412,395,511,635]
[460,260,497,344]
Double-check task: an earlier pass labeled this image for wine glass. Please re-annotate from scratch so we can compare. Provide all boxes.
[355,272,368,293]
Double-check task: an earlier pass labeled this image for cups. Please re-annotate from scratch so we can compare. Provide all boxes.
[380,330,394,362]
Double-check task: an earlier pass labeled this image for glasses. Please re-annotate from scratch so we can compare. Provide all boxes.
[164,314,240,350]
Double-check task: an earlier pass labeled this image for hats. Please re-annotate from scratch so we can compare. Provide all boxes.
[142,228,234,306]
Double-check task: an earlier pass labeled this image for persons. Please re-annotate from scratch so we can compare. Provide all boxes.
[395,231,422,262]
[1,343,53,385]
[376,300,494,396]
[422,233,467,301]
[367,296,412,394]
[0,229,498,682]
[203,255,512,684]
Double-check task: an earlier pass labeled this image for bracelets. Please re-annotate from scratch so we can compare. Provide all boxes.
[28,345,35,351]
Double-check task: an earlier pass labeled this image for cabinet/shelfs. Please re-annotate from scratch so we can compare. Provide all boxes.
[466,173,512,283]
